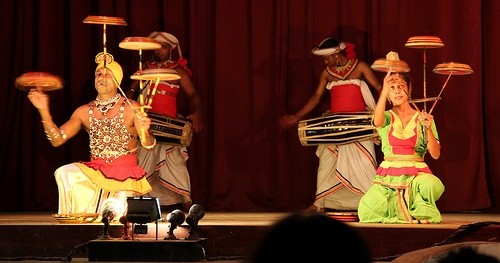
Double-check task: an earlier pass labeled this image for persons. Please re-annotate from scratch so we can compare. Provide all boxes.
[28,53,155,222]
[358,68,445,224]
[279,36,391,213]
[437,246,499,263]
[250,214,371,263]
[126,32,203,212]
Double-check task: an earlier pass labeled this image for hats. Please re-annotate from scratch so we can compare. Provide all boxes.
[149,31,182,58]
[94,52,123,87]
[310,37,347,57]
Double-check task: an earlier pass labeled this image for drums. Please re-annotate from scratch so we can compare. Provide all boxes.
[297,112,383,146]
[137,112,194,145]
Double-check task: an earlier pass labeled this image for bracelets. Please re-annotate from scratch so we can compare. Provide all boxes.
[41,116,61,141]
